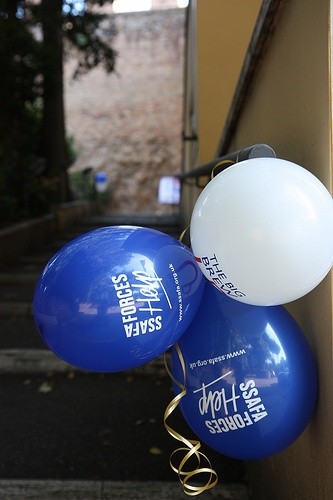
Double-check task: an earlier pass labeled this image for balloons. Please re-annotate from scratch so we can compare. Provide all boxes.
[34,224,208,373]
[168,282,318,460]
[190,156,332,307]
